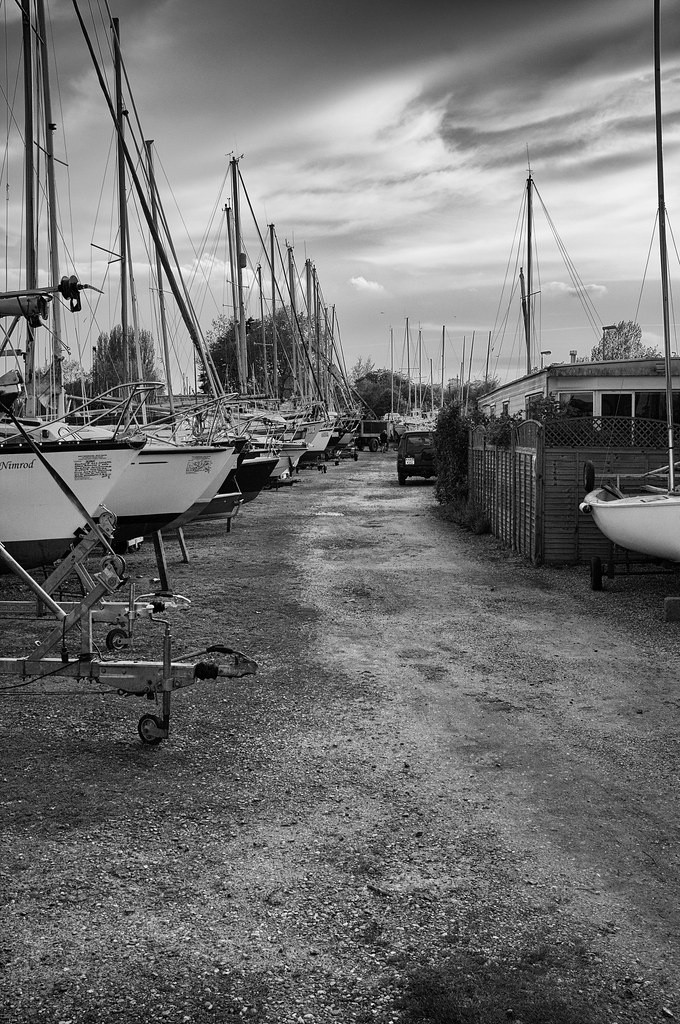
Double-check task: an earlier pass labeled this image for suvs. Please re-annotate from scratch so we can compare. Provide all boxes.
[393,429,439,486]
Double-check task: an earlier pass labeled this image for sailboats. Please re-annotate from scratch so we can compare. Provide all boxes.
[580,0,680,604]
[0,0,369,579]
[379,315,469,444]
